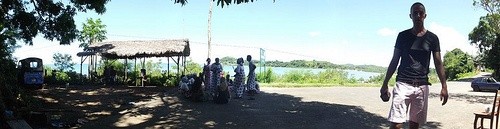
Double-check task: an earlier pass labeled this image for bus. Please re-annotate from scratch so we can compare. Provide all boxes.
[16,58,47,90]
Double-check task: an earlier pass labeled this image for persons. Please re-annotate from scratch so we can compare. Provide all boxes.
[178,71,204,98]
[203,58,216,97]
[211,58,223,98]
[103,64,147,87]
[234,57,246,99]
[246,54,261,96]
[215,72,233,104]
[380,2,450,129]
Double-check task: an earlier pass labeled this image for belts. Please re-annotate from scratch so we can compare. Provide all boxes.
[396,79,431,88]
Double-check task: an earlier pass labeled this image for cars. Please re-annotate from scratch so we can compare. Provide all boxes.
[471,76,500,92]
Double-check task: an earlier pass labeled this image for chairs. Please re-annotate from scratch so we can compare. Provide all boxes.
[473,89,500,129]
